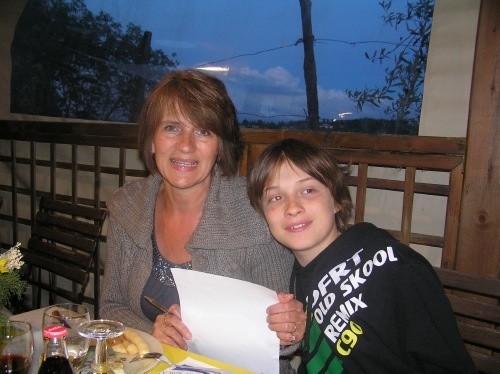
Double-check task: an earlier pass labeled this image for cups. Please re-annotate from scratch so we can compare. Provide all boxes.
[0,320,32,374]
[41,303,90,369]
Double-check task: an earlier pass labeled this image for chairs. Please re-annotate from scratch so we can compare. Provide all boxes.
[25,191,109,312]
[429,264,500,374]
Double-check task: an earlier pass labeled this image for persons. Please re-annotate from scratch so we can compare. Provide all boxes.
[98,68,307,374]
[246,138,480,374]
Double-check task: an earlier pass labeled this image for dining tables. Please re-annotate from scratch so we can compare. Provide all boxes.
[8,305,248,374]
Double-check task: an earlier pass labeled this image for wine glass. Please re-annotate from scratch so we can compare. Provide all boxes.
[77,319,125,374]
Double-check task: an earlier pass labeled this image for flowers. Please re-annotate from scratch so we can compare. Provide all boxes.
[0,243,28,310]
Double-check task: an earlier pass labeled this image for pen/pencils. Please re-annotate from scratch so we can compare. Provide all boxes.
[144,295,169,313]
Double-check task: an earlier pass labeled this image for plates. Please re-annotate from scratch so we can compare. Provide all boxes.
[4,322,163,373]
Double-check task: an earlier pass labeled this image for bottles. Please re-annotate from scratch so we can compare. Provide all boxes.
[38,325,73,374]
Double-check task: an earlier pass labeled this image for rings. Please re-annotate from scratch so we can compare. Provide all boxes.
[291,334,295,343]
[292,323,297,333]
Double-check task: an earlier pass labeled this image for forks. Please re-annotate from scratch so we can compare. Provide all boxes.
[129,352,229,374]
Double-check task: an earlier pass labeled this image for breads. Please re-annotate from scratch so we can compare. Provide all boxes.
[84,331,149,356]
